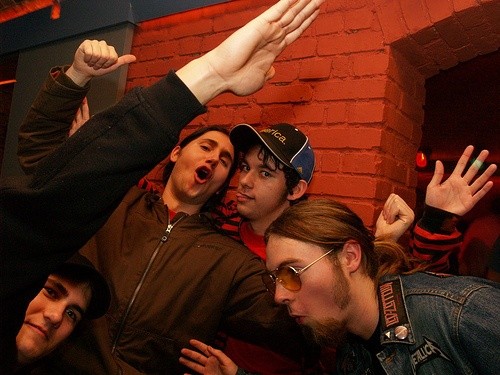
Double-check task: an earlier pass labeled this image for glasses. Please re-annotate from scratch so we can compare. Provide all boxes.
[262,244,340,296]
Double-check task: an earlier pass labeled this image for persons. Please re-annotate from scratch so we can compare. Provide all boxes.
[16,40,415,375]
[180,199,500,375]
[69,96,497,375]
[0,0,327,375]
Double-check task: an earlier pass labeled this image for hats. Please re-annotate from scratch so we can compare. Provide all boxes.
[230,122,316,185]
